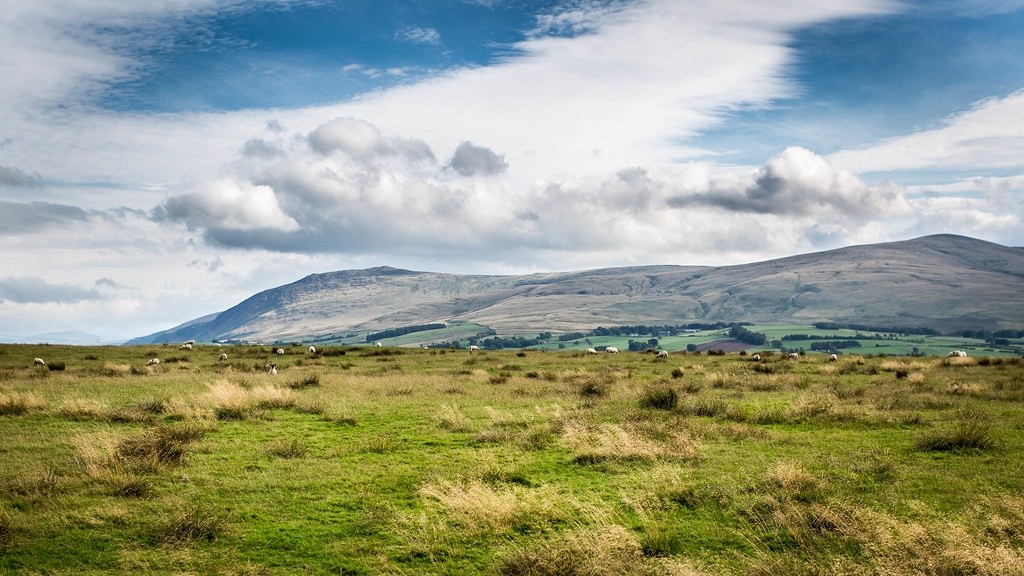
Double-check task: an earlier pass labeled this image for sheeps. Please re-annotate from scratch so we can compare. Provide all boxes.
[269,363,277,375]
[469,346,480,351]
[277,349,284,356]
[786,353,798,360]
[961,351,967,357]
[424,347,428,349]
[185,339,195,344]
[219,353,227,362]
[181,344,192,350]
[587,348,598,354]
[946,351,963,357]
[33,358,45,366]
[309,346,316,354]
[753,354,760,361]
[376,342,382,348]
[829,353,837,362]
[606,347,619,353]
[656,350,668,359]
[147,358,159,366]
